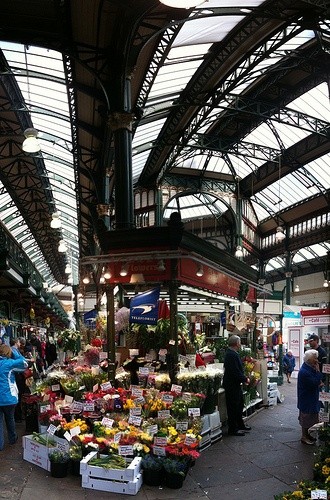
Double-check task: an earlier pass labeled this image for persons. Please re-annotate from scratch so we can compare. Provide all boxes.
[224,334,252,436]
[308,334,327,383]
[296,348,323,446]
[10,332,58,424]
[283,351,296,383]
[0,344,29,451]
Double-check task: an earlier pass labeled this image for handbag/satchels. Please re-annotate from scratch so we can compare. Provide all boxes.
[20,369,32,379]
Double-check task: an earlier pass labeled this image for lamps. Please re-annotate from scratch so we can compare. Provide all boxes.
[156,259,166,272]
[83,261,131,284]
[323,280,330,287]
[50,141,60,229]
[196,265,204,277]
[276,124,285,240]
[294,252,300,292]
[23,43,42,153]
[58,237,68,253]
[67,276,75,283]
[64,264,72,274]
[235,141,243,257]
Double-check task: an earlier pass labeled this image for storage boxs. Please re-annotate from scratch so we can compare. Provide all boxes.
[267,368,280,406]
[21,430,142,495]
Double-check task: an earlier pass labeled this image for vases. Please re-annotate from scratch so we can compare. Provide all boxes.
[25,384,260,488]
[313,468,325,482]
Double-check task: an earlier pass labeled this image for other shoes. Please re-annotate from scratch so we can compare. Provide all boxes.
[227,424,251,435]
[7,435,18,445]
[300,431,315,445]
[287,381,292,384]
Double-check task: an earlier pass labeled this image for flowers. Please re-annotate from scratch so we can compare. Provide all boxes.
[26,338,259,473]
[273,455,330,500]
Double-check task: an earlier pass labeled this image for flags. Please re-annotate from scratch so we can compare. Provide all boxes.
[130,287,160,326]
[84,310,96,329]
[226,307,235,324]
